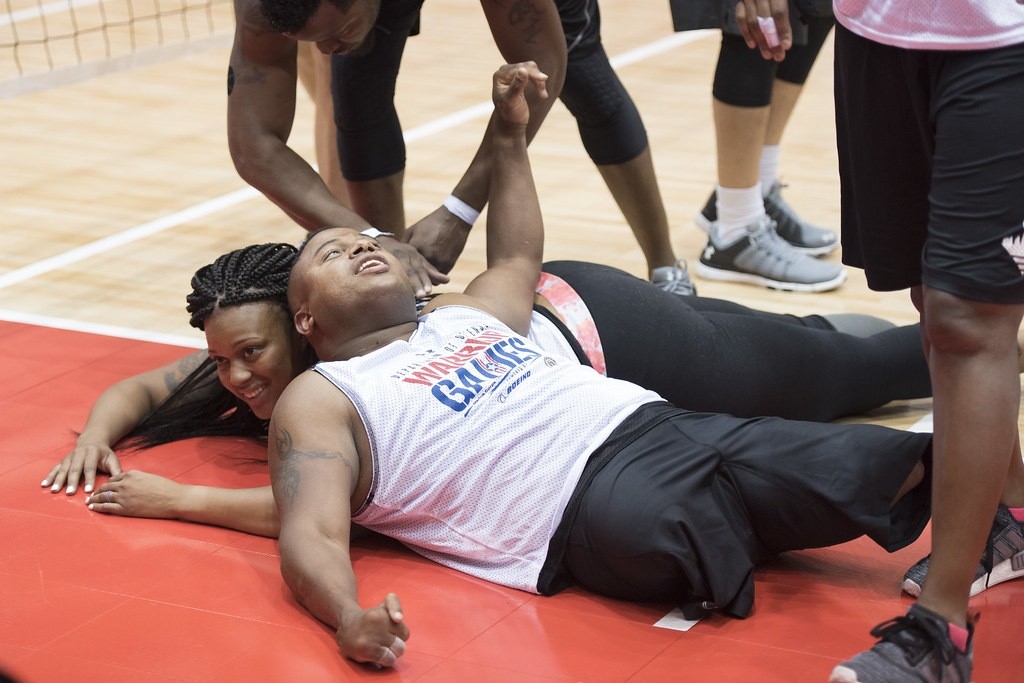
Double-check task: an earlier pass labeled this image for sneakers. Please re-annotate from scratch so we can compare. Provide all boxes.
[902,504,1024,598]
[697,213,848,292]
[697,176,841,256]
[829,603,981,683]
[650,258,697,296]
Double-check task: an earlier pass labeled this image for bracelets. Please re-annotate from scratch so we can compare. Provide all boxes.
[361,227,395,238]
[442,193,481,225]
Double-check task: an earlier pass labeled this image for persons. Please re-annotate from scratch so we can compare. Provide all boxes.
[41,0,1024,683]
